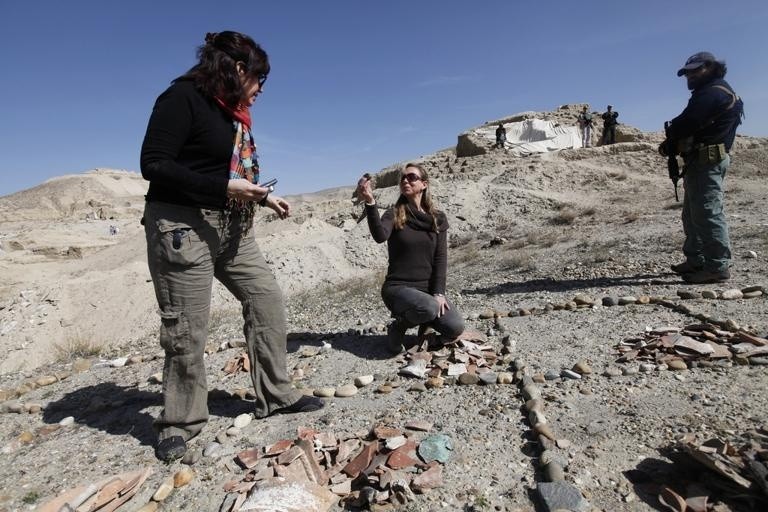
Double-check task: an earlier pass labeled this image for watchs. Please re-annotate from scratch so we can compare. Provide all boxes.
[432,293,445,297]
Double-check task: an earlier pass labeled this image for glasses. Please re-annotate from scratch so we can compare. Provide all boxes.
[401,172,423,182]
[256,70,267,88]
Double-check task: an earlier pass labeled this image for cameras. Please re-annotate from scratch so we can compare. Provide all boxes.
[258,178,278,192]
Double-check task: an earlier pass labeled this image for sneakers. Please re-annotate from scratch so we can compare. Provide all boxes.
[273,394,325,414]
[385,320,407,354]
[155,434,188,462]
[669,258,731,284]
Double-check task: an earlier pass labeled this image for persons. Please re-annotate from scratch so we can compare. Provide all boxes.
[602,104,618,145]
[658,51,746,286]
[496,123,507,149]
[578,106,593,148]
[140,30,324,462]
[356,163,466,354]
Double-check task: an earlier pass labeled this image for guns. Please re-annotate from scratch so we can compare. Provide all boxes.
[664,119,680,202]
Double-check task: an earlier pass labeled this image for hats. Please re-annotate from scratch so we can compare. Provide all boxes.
[676,50,717,78]
[607,104,614,108]
[583,105,589,109]
